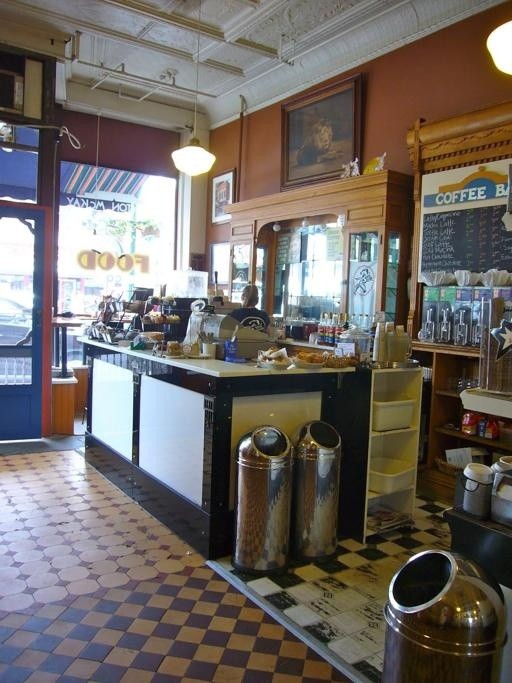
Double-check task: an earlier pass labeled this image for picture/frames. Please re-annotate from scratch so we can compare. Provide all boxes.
[208,165,239,227]
[278,69,368,194]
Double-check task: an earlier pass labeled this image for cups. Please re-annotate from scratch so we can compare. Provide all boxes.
[384,334,414,362]
[285,319,318,338]
[202,342,217,359]
[225,335,238,360]
[373,320,405,367]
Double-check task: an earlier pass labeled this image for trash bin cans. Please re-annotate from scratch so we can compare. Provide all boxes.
[291,421,341,562]
[381,549,508,683]
[232,424,295,575]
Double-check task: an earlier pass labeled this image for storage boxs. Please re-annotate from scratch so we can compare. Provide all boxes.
[367,384,417,433]
[365,449,415,494]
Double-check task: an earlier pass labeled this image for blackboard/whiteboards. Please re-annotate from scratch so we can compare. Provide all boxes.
[421,204,512,274]
[273,231,302,264]
[325,226,356,260]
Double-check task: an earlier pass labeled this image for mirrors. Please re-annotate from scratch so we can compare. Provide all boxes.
[271,222,346,323]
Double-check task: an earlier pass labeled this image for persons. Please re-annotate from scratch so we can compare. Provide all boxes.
[230,285,273,337]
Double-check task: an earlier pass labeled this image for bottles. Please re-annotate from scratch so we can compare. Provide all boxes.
[422,306,481,347]
[456,377,479,395]
[317,312,376,357]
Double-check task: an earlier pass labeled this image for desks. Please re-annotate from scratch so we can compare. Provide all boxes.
[442,504,511,590]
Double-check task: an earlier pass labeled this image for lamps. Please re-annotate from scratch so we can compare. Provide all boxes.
[164,0,220,176]
[271,217,285,232]
[300,214,311,228]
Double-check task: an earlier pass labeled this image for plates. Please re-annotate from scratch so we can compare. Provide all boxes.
[185,353,210,358]
[292,358,325,367]
[163,354,184,358]
[260,361,292,369]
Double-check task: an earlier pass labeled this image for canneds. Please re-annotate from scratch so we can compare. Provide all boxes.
[302,321,317,340]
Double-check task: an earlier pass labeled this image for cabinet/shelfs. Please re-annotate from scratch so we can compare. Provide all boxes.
[346,357,426,543]
[221,166,411,334]
[413,339,511,488]
